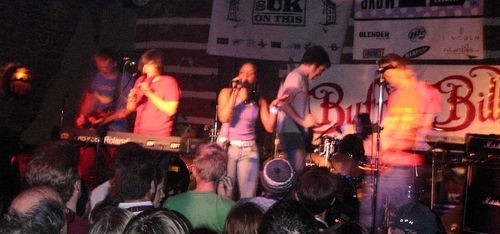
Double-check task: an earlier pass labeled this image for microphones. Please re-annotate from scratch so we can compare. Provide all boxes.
[132,60,139,76]
[236,80,242,84]
[376,61,398,73]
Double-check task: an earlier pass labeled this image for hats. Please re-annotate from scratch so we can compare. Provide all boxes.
[391,202,437,233]
[261,156,296,192]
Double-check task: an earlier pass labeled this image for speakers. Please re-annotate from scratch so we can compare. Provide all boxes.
[460,163,500,234]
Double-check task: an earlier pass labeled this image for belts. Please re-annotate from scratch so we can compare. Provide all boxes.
[217,137,256,146]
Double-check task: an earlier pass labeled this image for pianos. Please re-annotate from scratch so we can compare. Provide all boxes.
[58,123,206,154]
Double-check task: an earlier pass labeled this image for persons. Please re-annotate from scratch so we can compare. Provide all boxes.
[276,45,331,173]
[369,52,442,223]
[225,157,366,234]
[90,143,190,234]
[74,47,136,132]
[0,185,67,234]
[162,142,236,234]
[25,141,92,234]
[218,61,289,199]
[387,203,446,234]
[127,48,180,137]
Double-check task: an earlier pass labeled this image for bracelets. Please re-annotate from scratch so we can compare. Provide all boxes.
[269,105,278,113]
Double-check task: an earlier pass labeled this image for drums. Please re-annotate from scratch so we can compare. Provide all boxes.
[359,166,378,173]
